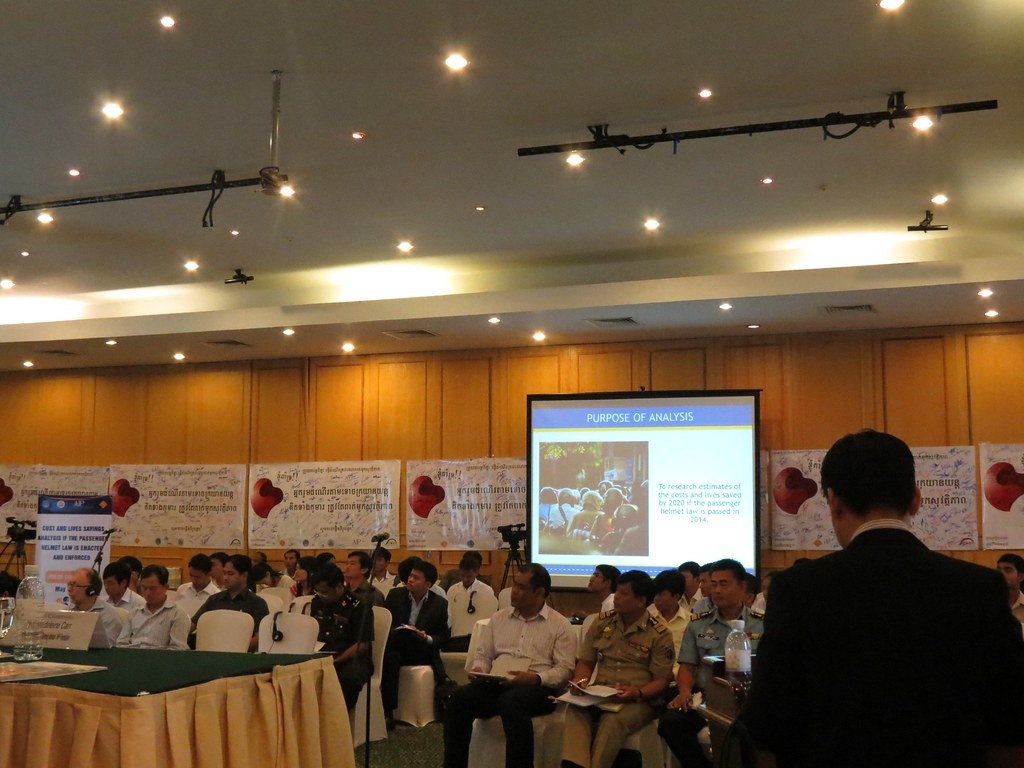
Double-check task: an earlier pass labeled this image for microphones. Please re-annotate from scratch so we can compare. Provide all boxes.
[498,523,525,533]
[103,527,117,534]
[371,532,389,542]
[6,518,25,524]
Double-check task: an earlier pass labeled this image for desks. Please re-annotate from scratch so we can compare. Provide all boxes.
[0,646,357,768]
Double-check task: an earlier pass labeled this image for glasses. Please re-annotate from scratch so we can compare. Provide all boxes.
[66,583,91,590]
[312,588,335,598]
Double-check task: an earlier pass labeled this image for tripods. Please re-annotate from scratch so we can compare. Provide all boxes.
[0,538,28,580]
[499,544,524,592]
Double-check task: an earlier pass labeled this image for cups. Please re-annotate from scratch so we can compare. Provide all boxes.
[0,597,15,639]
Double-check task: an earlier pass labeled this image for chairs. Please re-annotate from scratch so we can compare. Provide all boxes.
[168,587,681,768]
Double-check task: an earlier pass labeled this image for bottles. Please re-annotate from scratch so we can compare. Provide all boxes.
[13,565,45,661]
[725,620,752,708]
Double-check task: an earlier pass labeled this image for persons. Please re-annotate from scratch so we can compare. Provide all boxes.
[657,559,766,768]
[539,480,649,556]
[443,563,578,768]
[102,547,498,637]
[381,561,451,731]
[560,570,676,768]
[997,553,1024,641]
[187,554,270,651]
[0,570,22,599]
[311,563,374,713]
[732,431,1024,768]
[66,567,122,648]
[571,557,811,687]
[116,564,191,651]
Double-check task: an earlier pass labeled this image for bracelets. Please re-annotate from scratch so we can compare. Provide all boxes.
[638,689,643,697]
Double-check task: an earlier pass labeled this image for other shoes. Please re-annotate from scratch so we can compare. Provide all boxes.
[386,717,396,730]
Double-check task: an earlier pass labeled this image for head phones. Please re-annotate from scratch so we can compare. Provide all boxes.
[468,590,477,614]
[86,568,101,597]
[272,610,284,642]
